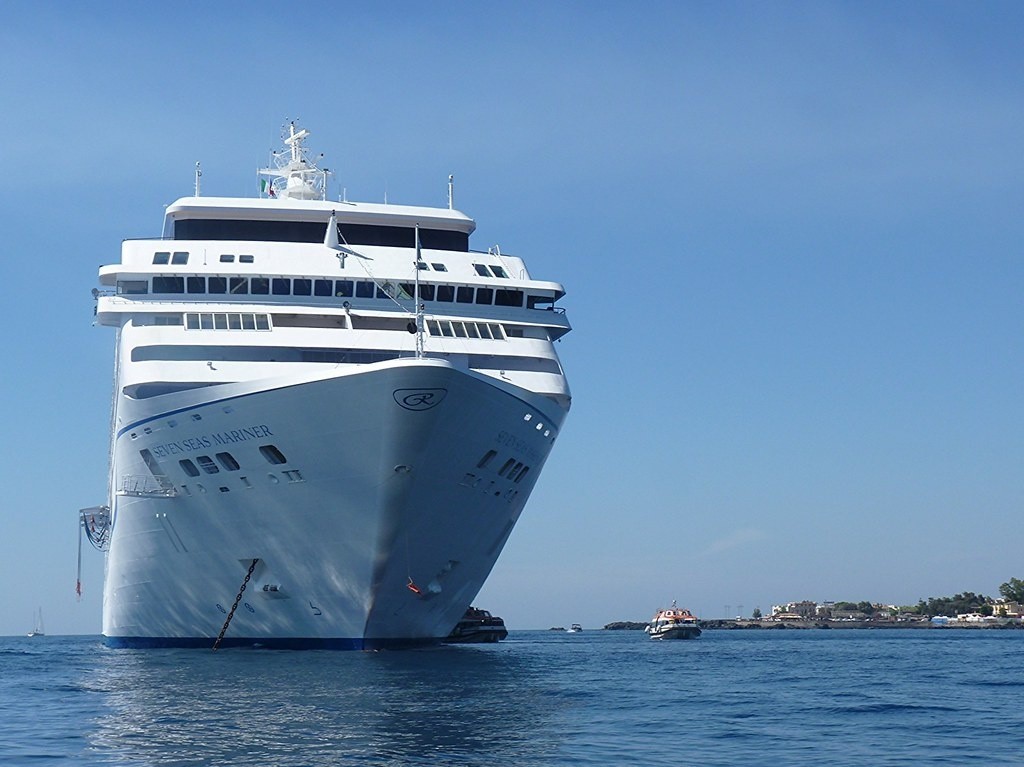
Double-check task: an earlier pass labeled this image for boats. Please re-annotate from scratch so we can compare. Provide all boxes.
[570,623,583,634]
[443,605,509,644]
[643,599,702,640]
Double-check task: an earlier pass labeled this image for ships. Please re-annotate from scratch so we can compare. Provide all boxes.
[79,114,572,654]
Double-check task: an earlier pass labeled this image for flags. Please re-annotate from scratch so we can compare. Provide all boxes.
[270,179,277,198]
[260,178,268,192]
